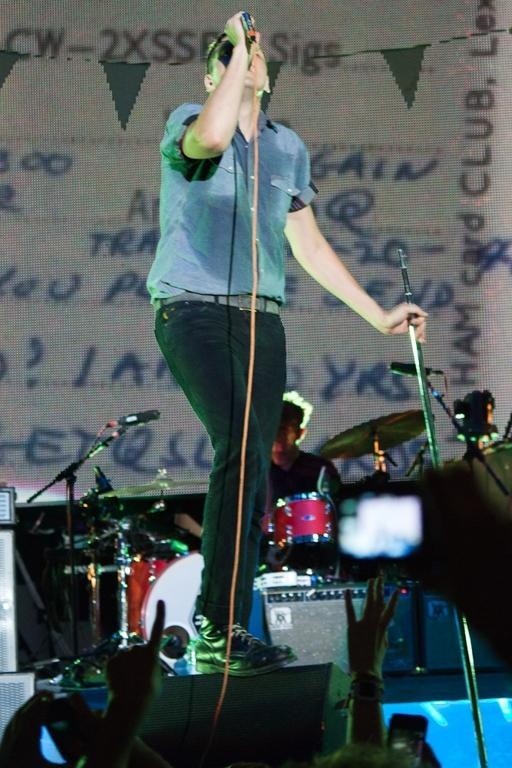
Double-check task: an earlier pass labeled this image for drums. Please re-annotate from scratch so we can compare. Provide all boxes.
[271,492,336,550]
[125,547,199,667]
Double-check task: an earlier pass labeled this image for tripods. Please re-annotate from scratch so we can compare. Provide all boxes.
[23,429,181,681]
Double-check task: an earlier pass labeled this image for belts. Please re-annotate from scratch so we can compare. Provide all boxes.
[154,293,280,314]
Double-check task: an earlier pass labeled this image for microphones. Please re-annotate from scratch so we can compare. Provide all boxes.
[240,11,257,44]
[388,362,431,378]
[118,408,161,426]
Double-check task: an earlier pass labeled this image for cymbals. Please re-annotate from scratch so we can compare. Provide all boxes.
[319,410,434,458]
[97,478,210,500]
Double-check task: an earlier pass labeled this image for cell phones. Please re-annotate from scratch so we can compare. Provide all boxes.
[44,697,86,762]
[387,713,427,768]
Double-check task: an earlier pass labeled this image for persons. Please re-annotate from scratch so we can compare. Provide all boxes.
[257,391,345,569]
[145,11,430,678]
[84,599,170,768]
[307,576,421,767]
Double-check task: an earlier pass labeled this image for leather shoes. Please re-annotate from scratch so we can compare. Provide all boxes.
[194,623,297,676]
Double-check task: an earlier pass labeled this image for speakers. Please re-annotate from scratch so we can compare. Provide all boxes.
[260,579,416,674]
[135,659,351,767]
[420,588,505,671]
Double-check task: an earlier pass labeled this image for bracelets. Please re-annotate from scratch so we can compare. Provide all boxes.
[344,670,384,708]
